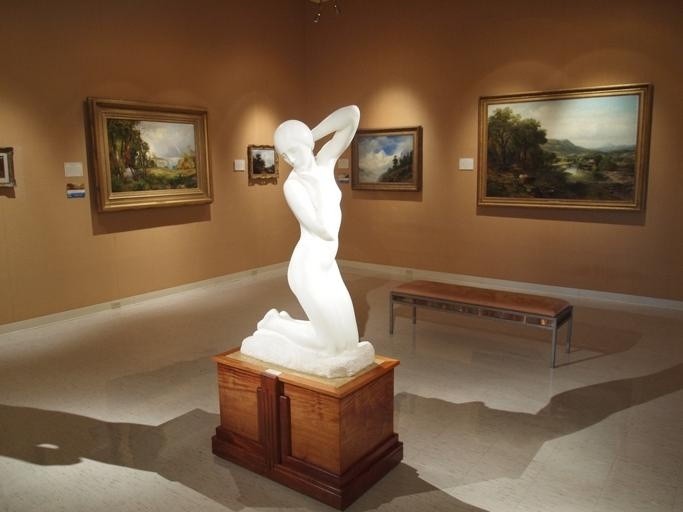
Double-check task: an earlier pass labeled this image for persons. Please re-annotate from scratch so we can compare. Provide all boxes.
[256,105,361,354]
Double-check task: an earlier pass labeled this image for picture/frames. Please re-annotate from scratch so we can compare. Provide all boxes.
[0,147,16,199]
[88,98,213,212]
[475,81,655,227]
[351,125,423,191]
[247,144,280,186]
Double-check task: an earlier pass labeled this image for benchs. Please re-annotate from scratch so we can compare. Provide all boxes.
[389,279,575,369]
